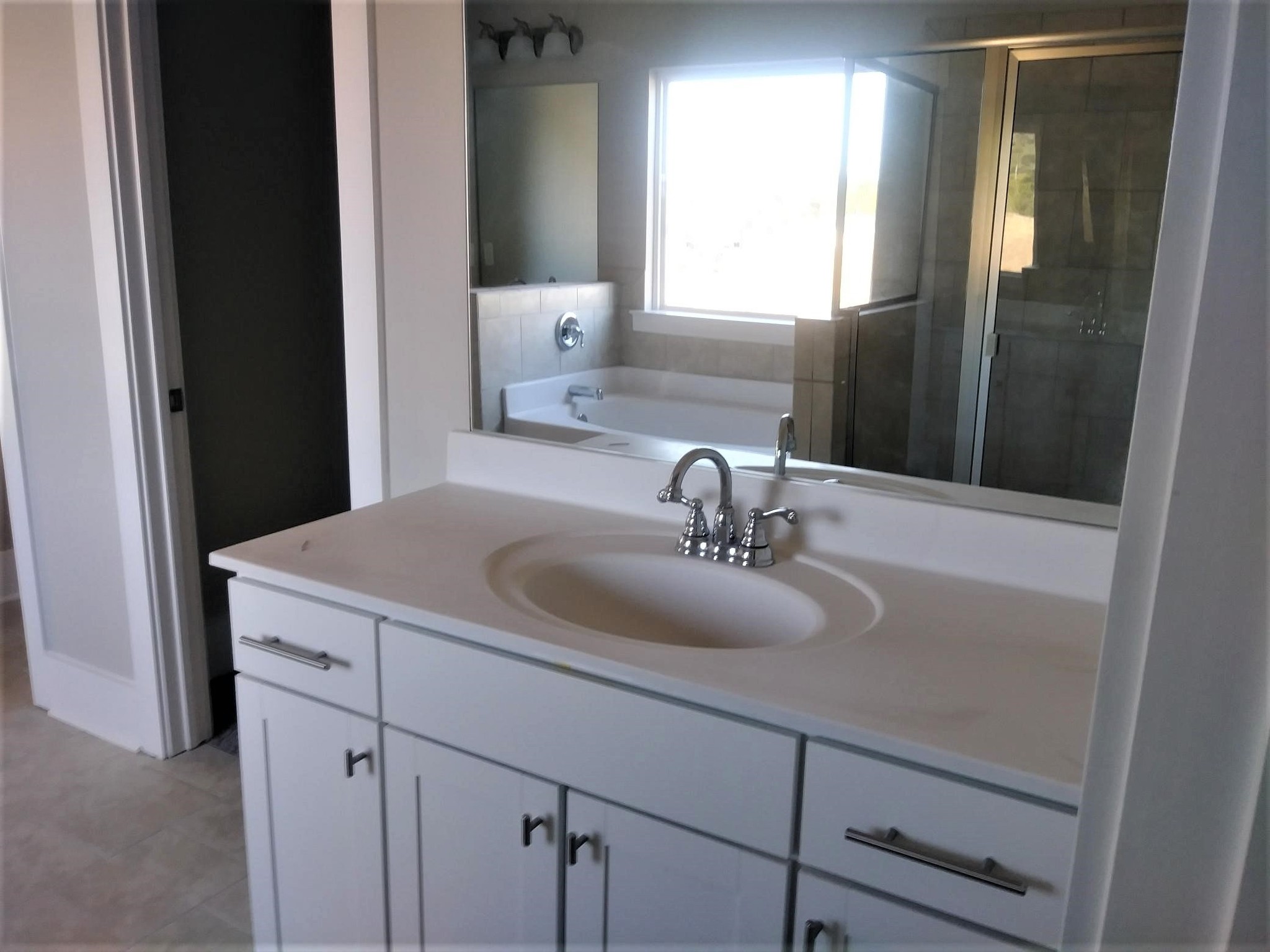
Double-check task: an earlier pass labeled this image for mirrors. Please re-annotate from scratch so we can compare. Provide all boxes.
[455,0,1196,530]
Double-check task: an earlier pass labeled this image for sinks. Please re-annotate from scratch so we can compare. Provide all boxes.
[483,532,885,650]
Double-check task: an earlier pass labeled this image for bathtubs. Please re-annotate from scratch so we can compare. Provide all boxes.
[500,365,794,461]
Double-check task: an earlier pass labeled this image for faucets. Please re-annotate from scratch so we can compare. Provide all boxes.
[773,413,797,476]
[568,384,604,401]
[656,447,735,546]
